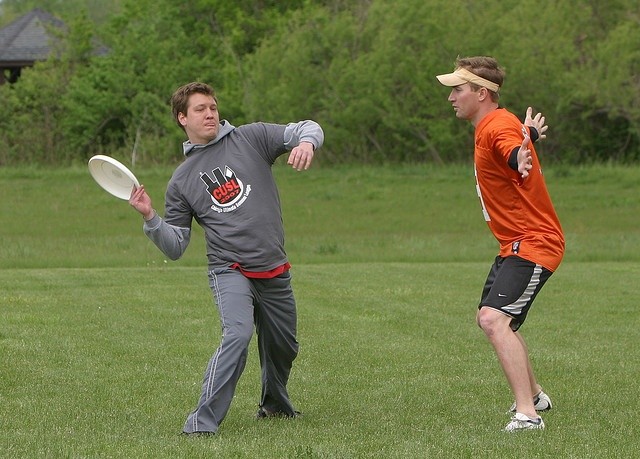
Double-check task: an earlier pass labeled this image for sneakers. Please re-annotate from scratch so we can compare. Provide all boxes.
[502,414,546,431]
[506,388,553,413]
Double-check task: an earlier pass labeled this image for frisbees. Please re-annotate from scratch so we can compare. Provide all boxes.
[88,154,140,200]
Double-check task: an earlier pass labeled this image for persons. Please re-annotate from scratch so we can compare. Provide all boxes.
[436,56,565,435]
[130,82,324,440]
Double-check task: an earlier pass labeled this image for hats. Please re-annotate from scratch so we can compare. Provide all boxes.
[435,65,501,93]
